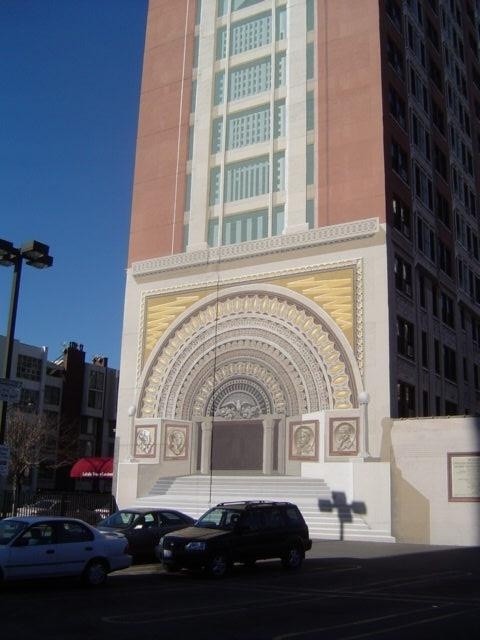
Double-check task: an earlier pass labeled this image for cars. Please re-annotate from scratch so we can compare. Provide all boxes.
[0,516,133,587]
[17,498,95,523]
[97,506,215,558]
[93,502,114,521]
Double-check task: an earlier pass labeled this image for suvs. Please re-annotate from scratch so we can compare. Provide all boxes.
[158,500,314,575]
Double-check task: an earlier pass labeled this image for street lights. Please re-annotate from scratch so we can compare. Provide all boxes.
[0,238,53,444]
